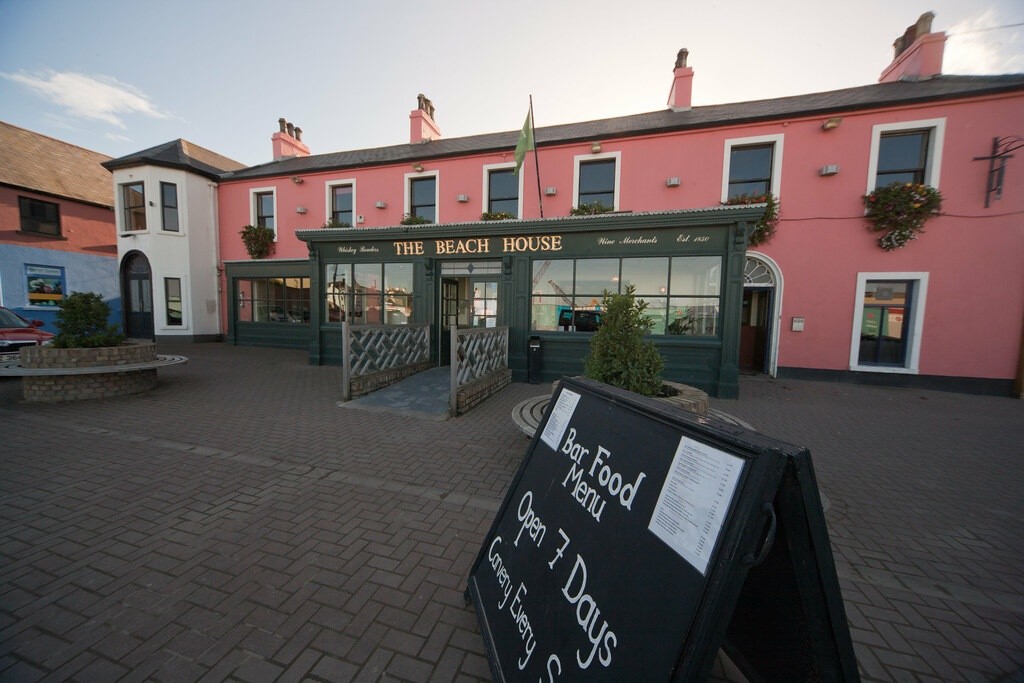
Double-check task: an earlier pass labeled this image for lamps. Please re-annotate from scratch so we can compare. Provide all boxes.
[412,163,424,171]
[290,175,303,184]
[592,142,600,152]
[823,118,843,129]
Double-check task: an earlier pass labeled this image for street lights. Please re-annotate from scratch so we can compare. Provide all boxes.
[333,274,346,306]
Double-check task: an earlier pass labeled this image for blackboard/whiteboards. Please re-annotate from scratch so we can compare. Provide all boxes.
[467,374,774,683]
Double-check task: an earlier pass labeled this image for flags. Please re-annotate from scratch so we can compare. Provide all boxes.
[512,105,534,175]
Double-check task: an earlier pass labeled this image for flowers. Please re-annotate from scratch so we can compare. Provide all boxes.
[570,199,613,216]
[480,212,518,221]
[320,217,353,228]
[861,181,944,250]
[400,212,432,225]
[720,191,785,248]
[238,225,274,259]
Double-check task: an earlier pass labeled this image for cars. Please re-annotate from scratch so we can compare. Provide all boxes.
[258,305,310,323]
[0,307,58,362]
[329,301,346,322]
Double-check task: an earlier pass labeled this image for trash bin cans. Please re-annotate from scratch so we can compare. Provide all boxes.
[526,335,542,383]
